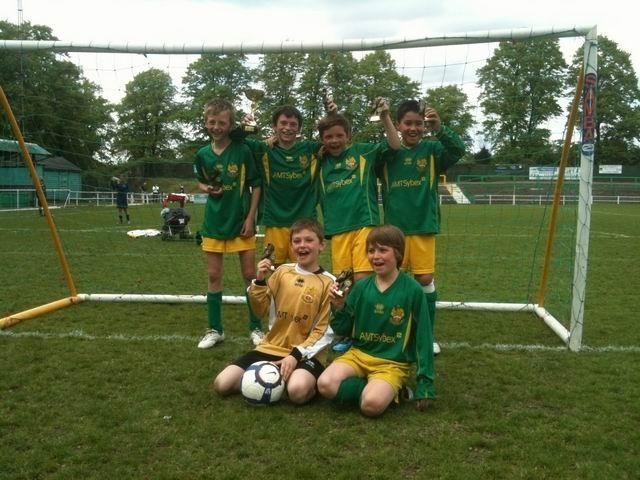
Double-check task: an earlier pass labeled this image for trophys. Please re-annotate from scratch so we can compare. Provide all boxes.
[242,87,265,136]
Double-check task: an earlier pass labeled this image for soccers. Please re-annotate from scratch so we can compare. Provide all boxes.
[241,360,286,406]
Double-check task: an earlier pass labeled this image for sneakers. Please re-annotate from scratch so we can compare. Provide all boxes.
[400,385,414,403]
[434,342,441,354]
[333,337,353,351]
[250,327,265,346]
[198,328,225,349]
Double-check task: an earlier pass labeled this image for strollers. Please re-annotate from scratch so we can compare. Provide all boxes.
[159,191,194,242]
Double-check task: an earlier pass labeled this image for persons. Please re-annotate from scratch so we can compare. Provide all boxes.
[212,215,337,406]
[157,206,198,243]
[191,98,266,350]
[262,96,404,290]
[151,183,160,204]
[178,183,185,206]
[35,177,47,218]
[112,172,131,223]
[227,96,339,275]
[367,94,466,357]
[139,182,148,198]
[314,221,437,420]
